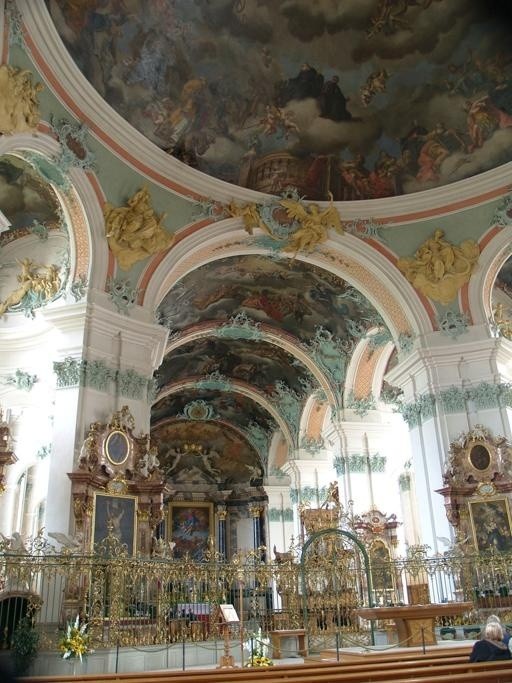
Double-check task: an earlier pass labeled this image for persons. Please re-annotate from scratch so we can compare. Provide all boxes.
[416,226,456,279]
[12,254,63,299]
[162,447,190,475]
[197,447,222,475]
[506,623,512,658]
[103,182,155,240]
[103,499,126,544]
[285,186,334,266]
[468,621,512,663]
[480,613,510,646]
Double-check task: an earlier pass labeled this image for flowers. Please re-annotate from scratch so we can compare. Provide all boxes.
[57,615,94,664]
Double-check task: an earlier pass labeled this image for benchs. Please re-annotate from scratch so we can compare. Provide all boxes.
[0,651,512,683]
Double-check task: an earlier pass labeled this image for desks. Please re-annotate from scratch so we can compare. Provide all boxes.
[270,629,308,659]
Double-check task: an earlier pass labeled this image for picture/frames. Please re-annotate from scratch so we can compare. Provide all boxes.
[468,497,512,563]
[168,501,215,565]
[89,491,138,559]
